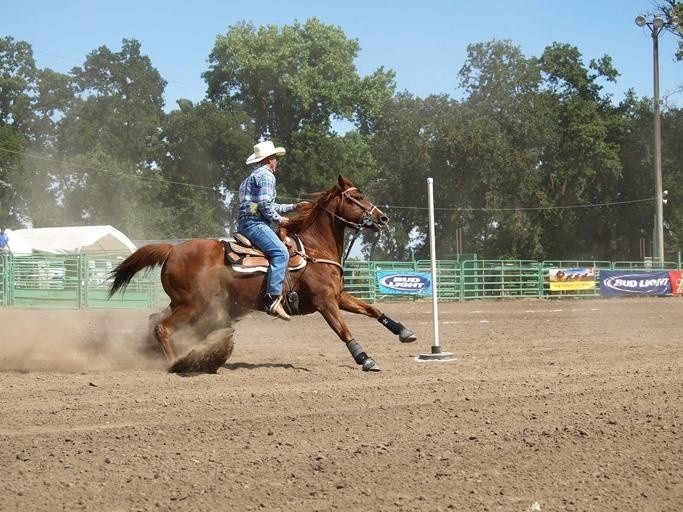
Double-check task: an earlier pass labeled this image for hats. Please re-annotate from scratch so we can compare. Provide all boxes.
[244,141,286,165]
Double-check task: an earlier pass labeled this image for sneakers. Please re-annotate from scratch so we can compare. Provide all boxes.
[265,302,291,322]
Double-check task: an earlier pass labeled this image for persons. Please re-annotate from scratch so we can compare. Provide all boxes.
[236,140,310,322]
[0,222,11,252]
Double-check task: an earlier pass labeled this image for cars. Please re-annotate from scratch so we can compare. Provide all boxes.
[347,260,553,299]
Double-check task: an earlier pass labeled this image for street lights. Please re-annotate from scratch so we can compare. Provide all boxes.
[633,10,678,273]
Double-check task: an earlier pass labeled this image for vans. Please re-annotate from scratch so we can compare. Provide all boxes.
[3,252,153,295]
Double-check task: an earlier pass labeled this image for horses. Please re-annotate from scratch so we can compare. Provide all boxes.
[102,174,418,372]
